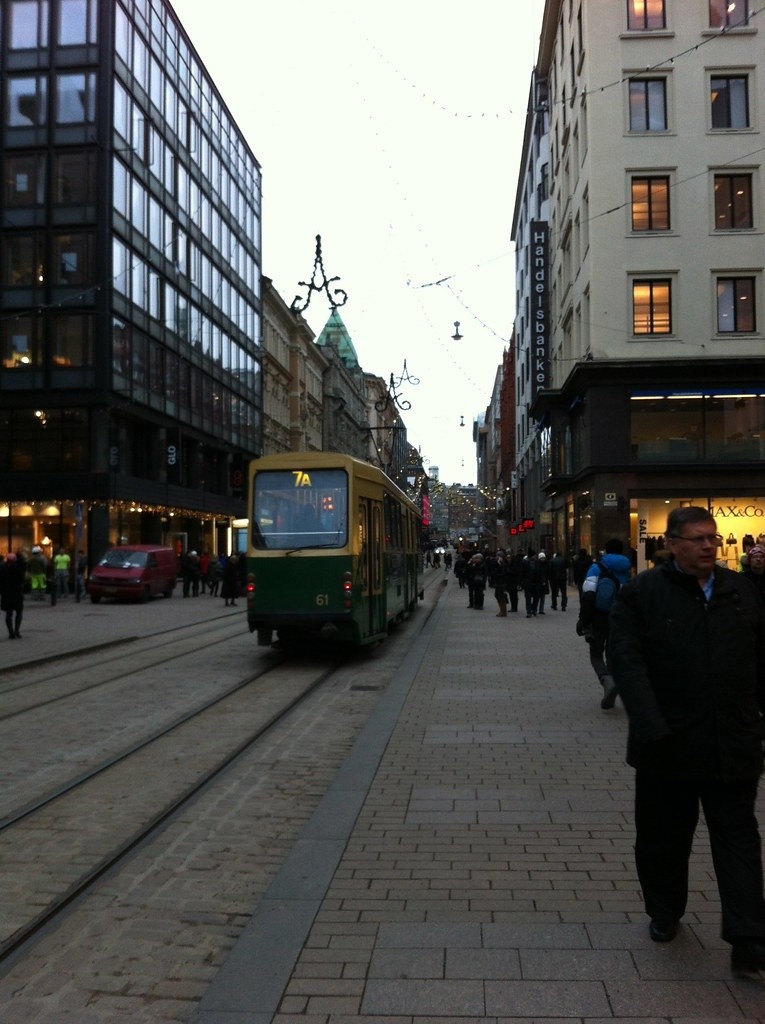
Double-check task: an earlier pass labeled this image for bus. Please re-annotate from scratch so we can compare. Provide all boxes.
[246,451,427,666]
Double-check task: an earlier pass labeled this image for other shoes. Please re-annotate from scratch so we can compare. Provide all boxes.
[57,593,69,598]
[182,590,237,606]
[75,597,87,602]
[9,633,21,639]
[509,606,566,617]
[33,596,45,601]
[467,603,484,610]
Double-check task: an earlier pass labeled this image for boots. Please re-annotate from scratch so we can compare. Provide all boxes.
[495,602,507,617]
[601,676,618,710]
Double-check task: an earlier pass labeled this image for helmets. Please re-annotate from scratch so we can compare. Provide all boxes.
[32,547,40,554]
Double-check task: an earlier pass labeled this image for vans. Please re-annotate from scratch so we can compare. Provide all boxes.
[86,545,179,605]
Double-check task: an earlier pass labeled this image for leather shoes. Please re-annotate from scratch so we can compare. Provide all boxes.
[729,947,764,980]
[651,916,683,943]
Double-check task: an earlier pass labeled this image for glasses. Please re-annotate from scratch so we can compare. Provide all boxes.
[668,534,722,546]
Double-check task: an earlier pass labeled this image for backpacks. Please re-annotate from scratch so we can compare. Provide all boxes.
[595,571,619,611]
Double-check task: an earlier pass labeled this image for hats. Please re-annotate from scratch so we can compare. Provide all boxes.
[7,553,16,563]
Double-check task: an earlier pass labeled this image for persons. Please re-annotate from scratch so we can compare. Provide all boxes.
[179,546,248,607]
[15,544,89,601]
[737,533,765,593]
[577,539,635,712]
[424,540,576,619]
[1,553,25,640]
[573,548,593,604]
[605,507,765,989]
[274,503,333,547]
